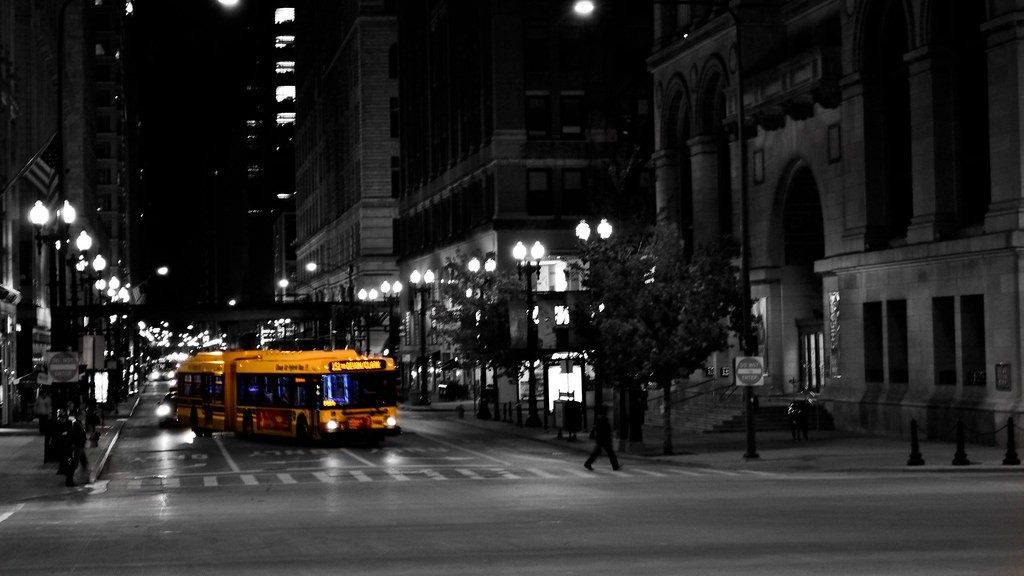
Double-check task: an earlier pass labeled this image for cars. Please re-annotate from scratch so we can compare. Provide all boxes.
[156,391,178,426]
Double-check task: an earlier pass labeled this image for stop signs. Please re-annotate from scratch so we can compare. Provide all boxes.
[44,352,79,383]
[735,356,764,386]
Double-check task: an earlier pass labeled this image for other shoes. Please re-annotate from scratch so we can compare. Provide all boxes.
[613,464,622,470]
[584,464,594,471]
[64,482,77,488]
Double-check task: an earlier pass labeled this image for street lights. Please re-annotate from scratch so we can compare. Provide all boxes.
[30,199,76,464]
[381,280,402,355]
[358,288,378,355]
[229,293,316,337]
[279,279,319,340]
[76,230,106,431]
[513,241,545,427]
[575,217,613,439]
[306,262,355,347]
[467,257,496,420]
[408,270,434,405]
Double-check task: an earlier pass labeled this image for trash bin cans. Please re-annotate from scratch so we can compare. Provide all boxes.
[552,400,582,442]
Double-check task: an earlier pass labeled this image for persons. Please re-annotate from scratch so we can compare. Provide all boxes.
[333,384,344,398]
[49,404,89,487]
[583,405,626,471]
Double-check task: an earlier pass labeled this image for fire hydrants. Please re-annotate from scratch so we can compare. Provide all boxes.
[455,404,465,419]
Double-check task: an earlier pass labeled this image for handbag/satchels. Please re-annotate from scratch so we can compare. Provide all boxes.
[589,426,596,440]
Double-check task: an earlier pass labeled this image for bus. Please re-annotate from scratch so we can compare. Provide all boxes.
[176,349,399,443]
